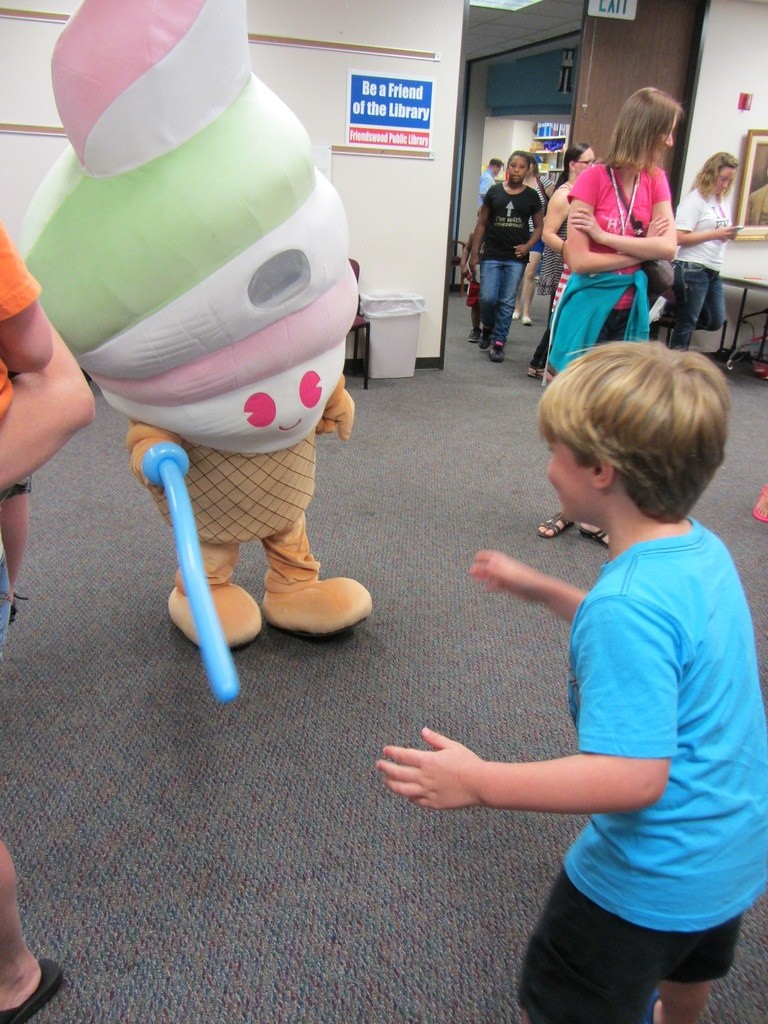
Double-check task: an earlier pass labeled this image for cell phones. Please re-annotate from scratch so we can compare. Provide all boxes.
[729,225,744,232]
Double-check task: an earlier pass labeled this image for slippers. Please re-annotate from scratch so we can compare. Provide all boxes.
[0,957,64,1024]
[753,483,768,523]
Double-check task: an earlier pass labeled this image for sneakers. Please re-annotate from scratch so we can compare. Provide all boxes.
[489,341,504,362]
[468,327,481,342]
[479,328,491,349]
[647,296,668,326]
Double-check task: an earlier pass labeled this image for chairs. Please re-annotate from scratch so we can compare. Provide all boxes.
[453,240,465,297]
[348,258,371,389]
[651,288,676,346]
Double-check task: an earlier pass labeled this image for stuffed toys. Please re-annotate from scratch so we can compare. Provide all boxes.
[18,0,372,652]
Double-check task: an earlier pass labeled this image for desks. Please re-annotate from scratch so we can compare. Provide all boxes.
[720,275,768,352]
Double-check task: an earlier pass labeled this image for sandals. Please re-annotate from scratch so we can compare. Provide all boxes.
[527,367,545,379]
[578,522,609,548]
[536,510,575,538]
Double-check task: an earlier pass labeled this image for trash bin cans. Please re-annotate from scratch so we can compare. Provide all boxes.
[360,288,425,379]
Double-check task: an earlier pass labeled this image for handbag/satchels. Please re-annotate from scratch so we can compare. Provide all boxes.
[465,281,481,310]
[644,259,674,292]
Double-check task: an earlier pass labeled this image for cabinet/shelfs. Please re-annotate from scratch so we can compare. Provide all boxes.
[530,135,567,186]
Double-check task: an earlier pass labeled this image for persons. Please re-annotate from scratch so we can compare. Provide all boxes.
[0,223,96,1024]
[374,338,768,1024]
[460,150,555,363]
[753,484,768,523]
[537,85,685,551]
[527,143,596,382]
[667,150,739,352]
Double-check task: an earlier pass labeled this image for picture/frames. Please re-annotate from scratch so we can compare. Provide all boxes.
[732,129,768,240]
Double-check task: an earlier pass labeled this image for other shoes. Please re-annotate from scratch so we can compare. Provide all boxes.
[512,312,522,319]
[521,316,532,326]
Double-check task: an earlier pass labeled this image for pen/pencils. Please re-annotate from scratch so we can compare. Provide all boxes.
[743,276,765,280]
